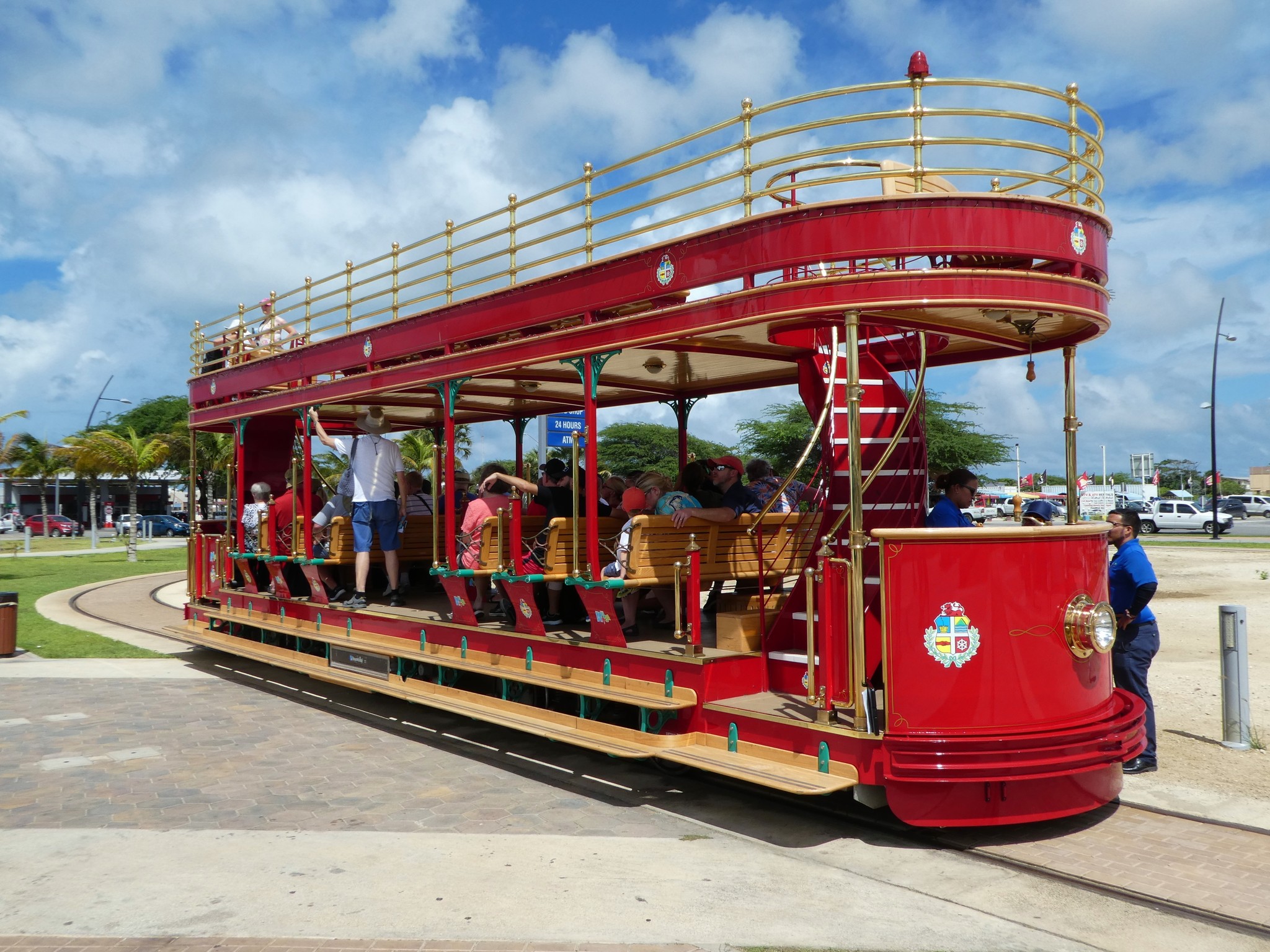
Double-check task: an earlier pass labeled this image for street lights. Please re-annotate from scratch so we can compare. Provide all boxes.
[77,374,132,538]
[1179,473,1185,500]
[1199,297,1237,541]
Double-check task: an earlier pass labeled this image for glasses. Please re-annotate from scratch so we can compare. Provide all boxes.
[959,484,977,496]
[1110,522,1129,527]
[713,465,733,471]
[602,483,613,490]
[541,472,548,476]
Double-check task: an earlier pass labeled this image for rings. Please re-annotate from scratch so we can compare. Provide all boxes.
[679,518,682,520]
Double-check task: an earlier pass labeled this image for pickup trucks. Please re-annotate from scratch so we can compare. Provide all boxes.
[959,507,986,523]
[990,497,1031,518]
[969,500,997,523]
[1137,500,1235,534]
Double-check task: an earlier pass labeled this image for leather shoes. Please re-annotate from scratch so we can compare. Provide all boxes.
[1122,758,1158,774]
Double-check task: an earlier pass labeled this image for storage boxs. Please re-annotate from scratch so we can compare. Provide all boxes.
[716,592,791,651]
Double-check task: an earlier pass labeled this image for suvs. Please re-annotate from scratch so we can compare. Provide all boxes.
[23,514,84,538]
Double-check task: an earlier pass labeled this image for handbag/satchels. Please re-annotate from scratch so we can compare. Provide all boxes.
[337,438,359,497]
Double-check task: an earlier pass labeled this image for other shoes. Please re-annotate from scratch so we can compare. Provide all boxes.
[268,580,445,608]
[703,591,721,611]
[652,619,675,630]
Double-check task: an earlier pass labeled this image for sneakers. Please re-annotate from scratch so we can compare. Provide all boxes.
[541,611,562,625]
[500,598,515,625]
[585,616,590,622]
[488,607,506,616]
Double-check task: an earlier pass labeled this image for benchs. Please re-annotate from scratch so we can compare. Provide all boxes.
[293,510,460,605]
[228,520,269,594]
[488,516,624,636]
[257,516,315,598]
[429,516,527,630]
[565,512,824,652]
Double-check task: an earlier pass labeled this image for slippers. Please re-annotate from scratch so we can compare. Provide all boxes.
[474,610,484,616]
[488,598,500,603]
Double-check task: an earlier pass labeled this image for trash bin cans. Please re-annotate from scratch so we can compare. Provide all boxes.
[0,592,19,655]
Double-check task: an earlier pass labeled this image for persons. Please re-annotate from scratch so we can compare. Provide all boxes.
[268,468,352,600]
[309,406,407,608]
[734,459,822,592]
[672,455,763,529]
[396,471,478,599]
[457,464,510,616]
[1022,501,1052,526]
[480,459,704,636]
[925,469,979,528]
[1105,508,1160,774]
[214,319,256,367]
[258,298,297,353]
[241,482,271,591]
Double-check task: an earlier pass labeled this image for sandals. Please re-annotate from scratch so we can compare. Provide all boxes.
[622,624,639,636]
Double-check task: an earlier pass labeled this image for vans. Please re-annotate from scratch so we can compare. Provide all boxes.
[1228,495,1270,519]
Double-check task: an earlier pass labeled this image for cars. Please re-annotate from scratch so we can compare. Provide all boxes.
[0,513,13,533]
[213,511,227,519]
[1038,499,1067,518]
[136,515,191,536]
[115,514,142,537]
[1020,501,1033,514]
[1114,497,1154,512]
[1204,499,1248,520]
[171,510,188,523]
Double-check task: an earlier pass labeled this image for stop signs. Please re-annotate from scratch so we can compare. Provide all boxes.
[106,508,112,514]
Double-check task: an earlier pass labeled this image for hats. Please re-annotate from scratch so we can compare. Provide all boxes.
[539,458,566,479]
[622,487,649,515]
[707,455,744,476]
[356,409,392,435]
[285,467,303,488]
[552,465,585,484]
[454,472,475,485]
[1024,500,1052,521]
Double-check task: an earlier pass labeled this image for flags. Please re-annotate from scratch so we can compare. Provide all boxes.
[1108,472,1114,489]
[1087,474,1095,485]
[1187,476,1193,488]
[1206,472,1220,485]
[1020,473,1033,487]
[1037,469,1046,485]
[1077,472,1087,489]
[1152,470,1159,485]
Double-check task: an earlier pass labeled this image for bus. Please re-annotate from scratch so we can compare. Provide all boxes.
[178,48,1150,827]
